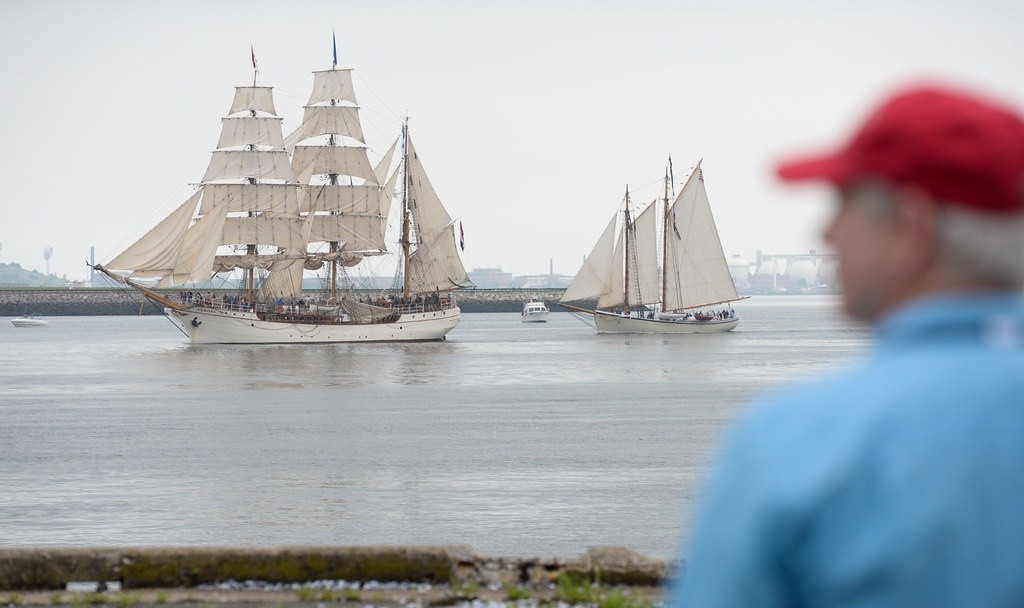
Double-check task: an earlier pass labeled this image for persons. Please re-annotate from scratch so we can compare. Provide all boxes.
[684,310,715,321]
[665,87,1024,608]
[626,309,630,315]
[298,298,304,305]
[647,312,654,319]
[359,290,452,314]
[180,289,246,311]
[610,307,622,314]
[715,309,735,320]
[277,298,284,304]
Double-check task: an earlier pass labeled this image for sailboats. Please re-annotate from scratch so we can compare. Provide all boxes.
[84,29,477,343]
[553,155,752,334]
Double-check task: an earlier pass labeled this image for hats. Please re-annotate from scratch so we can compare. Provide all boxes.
[773,84,1024,220]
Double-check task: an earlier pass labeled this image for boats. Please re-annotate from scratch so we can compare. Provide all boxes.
[521,299,550,322]
